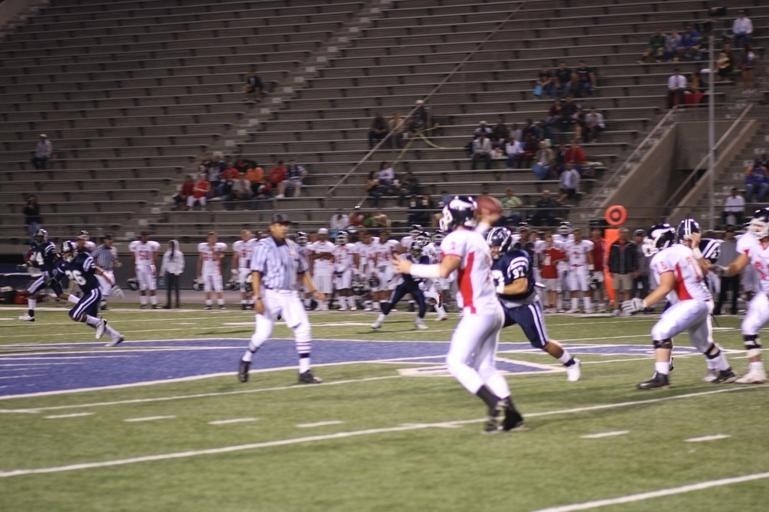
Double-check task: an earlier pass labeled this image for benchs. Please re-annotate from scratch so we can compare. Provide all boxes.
[0,2,769,275]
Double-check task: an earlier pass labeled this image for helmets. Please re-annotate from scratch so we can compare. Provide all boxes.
[32,229,49,246]
[296,228,349,247]
[642,219,701,257]
[61,230,113,254]
[743,208,769,240]
[409,225,447,252]
[484,221,572,260]
[439,196,478,236]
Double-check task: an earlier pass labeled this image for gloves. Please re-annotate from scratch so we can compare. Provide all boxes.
[622,298,646,313]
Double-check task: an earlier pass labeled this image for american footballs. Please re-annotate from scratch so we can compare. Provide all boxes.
[476,195,503,215]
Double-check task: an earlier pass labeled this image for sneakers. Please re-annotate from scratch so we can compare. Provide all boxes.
[637,358,673,390]
[19,313,35,321]
[482,397,524,435]
[96,319,124,347]
[702,367,768,383]
[566,359,581,381]
[299,370,322,383]
[239,360,250,382]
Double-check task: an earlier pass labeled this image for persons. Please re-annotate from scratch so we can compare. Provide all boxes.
[187,9,767,227]
[31,133,52,170]
[19,196,767,434]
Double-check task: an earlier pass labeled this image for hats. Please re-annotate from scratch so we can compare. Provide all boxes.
[271,212,299,225]
